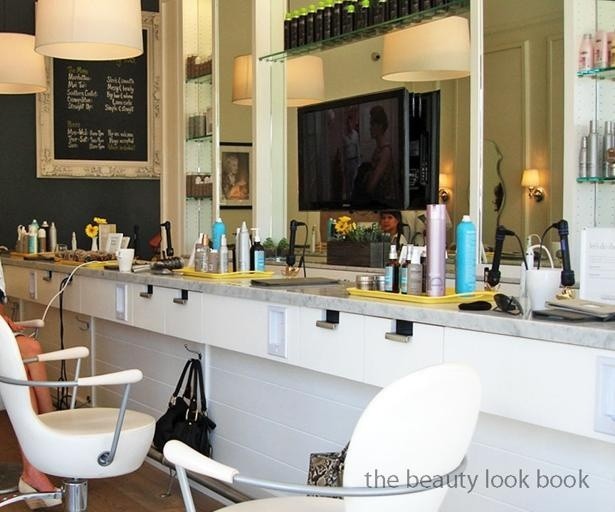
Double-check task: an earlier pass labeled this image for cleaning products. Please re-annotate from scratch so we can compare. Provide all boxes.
[192,216,265,273]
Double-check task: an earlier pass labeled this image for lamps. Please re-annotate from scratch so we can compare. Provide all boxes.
[232,53,253,105]
[380,15,472,82]
[520,169,546,202]
[34,0,145,61]
[0,32,48,94]
[287,55,325,108]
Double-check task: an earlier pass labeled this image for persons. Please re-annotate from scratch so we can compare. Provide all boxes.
[0,256,63,510]
[326,105,392,194]
[223,153,239,199]
[379,210,408,246]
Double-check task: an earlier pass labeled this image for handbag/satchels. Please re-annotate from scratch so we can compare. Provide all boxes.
[306,452,343,499]
[153,397,216,456]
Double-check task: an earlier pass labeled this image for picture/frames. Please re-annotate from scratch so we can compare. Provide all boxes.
[220,142,252,209]
[34,1,162,181]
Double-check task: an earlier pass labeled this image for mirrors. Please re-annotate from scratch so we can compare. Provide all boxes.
[470,0,596,289]
[212,0,271,245]
[271,9,470,279]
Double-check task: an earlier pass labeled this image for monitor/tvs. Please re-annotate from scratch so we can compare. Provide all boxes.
[411,91,439,210]
[297,87,411,210]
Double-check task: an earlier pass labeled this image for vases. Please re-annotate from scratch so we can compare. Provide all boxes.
[91,236,99,251]
[384,242,393,268]
[327,239,384,268]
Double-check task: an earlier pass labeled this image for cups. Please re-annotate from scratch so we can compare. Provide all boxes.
[524,268,564,311]
[115,248,134,274]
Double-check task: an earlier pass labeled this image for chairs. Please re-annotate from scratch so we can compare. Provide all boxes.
[162,361,483,512]
[0,315,156,512]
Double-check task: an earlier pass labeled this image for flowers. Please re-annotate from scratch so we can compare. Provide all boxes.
[84,216,108,238]
[331,215,384,242]
[381,232,391,242]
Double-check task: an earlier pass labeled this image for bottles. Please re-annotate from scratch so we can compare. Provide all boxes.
[577,121,614,178]
[326,218,337,243]
[426,203,446,297]
[185,51,213,78]
[187,111,206,138]
[579,30,614,76]
[281,0,466,52]
[194,215,251,274]
[71,231,77,251]
[185,171,215,197]
[454,214,477,296]
[309,225,317,255]
[386,243,427,297]
[16,220,59,255]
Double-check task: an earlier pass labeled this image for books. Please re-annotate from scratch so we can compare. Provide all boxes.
[532,298,615,323]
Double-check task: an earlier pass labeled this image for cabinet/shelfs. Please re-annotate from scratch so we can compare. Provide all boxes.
[576,66,615,184]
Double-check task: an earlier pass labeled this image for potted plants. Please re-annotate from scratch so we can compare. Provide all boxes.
[277,237,291,257]
[262,237,275,258]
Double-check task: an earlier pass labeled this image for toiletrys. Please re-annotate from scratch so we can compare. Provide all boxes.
[356,245,427,296]
[195,217,265,273]
[106,233,135,272]
[16,219,57,253]
[577,33,614,72]
[17,219,57,254]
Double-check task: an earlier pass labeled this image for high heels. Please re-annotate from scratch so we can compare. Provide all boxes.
[19,477,63,510]
